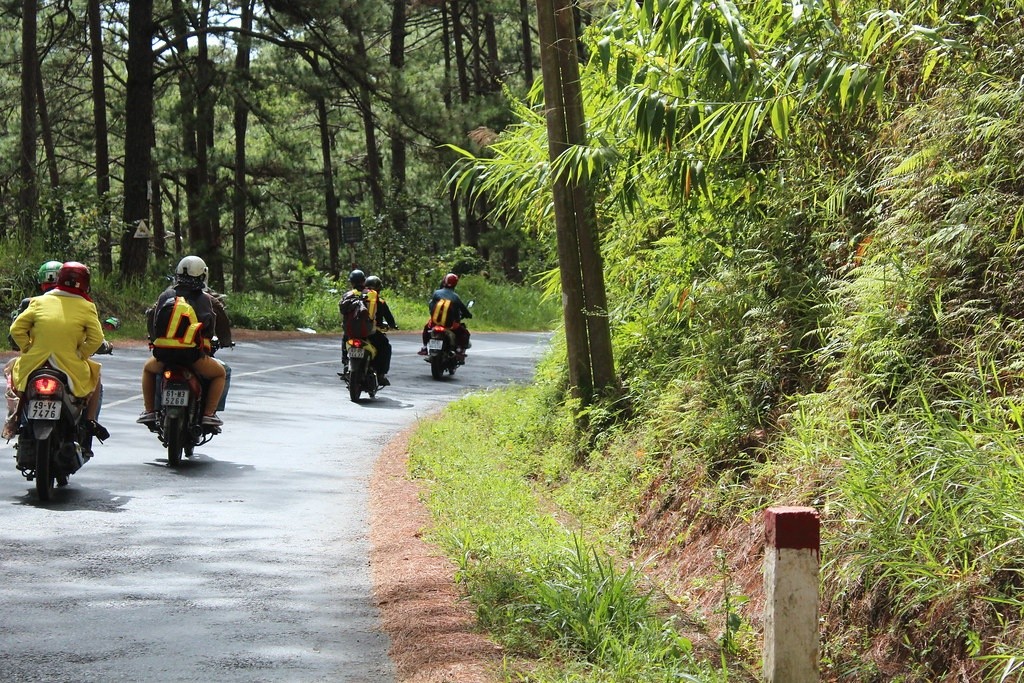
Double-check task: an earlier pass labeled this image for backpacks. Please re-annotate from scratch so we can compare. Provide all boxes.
[153,290,211,360]
[339,295,373,339]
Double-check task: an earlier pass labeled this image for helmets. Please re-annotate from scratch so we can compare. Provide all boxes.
[38,261,63,285]
[175,255,207,277]
[57,261,90,291]
[443,273,458,288]
[365,276,384,290]
[348,269,366,283]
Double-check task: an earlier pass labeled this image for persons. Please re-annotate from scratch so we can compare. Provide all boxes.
[417,273,470,365]
[339,270,396,386]
[9,260,113,420]
[137,255,233,426]
[2,262,109,444]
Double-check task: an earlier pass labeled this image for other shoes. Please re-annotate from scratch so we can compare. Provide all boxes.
[215,426,223,434]
[136,411,155,423]
[377,377,389,385]
[417,347,427,355]
[340,371,348,380]
[457,351,468,358]
[86,420,109,441]
[202,413,224,426]
[3,423,18,439]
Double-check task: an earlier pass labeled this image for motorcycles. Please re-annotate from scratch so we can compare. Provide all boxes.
[144,335,236,469]
[423,299,475,380]
[11,316,121,502]
[337,320,400,403]
